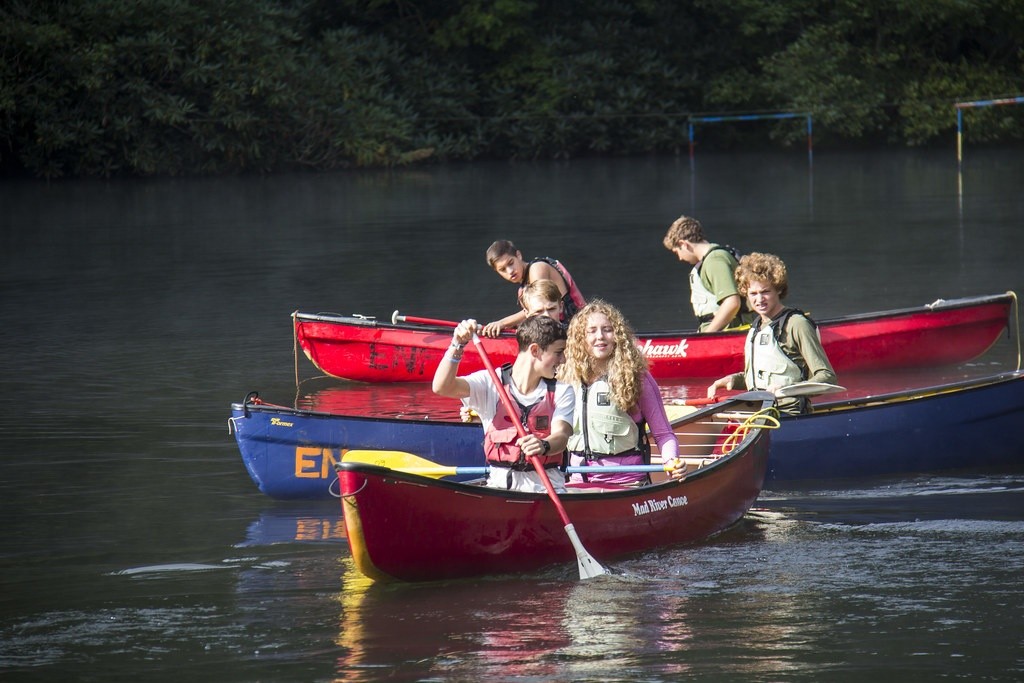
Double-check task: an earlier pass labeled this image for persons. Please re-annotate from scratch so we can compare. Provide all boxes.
[521,278,568,325]
[707,252,840,417]
[431,314,575,494]
[482,240,587,339]
[662,216,746,333]
[564,299,689,493]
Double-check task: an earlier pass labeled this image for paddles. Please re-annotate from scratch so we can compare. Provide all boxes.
[724,312,805,332]
[473,333,607,582]
[672,397,721,405]
[392,310,518,336]
[330,448,680,481]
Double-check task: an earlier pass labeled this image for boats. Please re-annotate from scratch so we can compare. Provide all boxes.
[291,286,1016,383]
[333,391,776,583]
[230,369,1023,503]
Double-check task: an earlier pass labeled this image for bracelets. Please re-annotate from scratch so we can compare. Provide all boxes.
[445,338,466,363]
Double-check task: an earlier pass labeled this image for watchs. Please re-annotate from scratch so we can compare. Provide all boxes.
[541,439,550,456]
